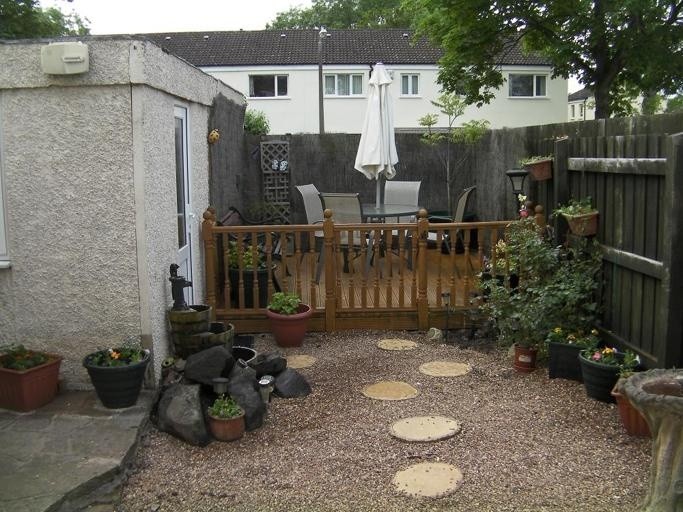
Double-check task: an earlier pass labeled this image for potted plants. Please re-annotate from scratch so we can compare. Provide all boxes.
[421,95,490,254]
[228,241,277,308]
[266,292,312,348]
[83,343,151,409]
[552,195,600,236]
[478,266,594,376]
[207,394,246,442]
[518,153,554,182]
[0,341,65,413]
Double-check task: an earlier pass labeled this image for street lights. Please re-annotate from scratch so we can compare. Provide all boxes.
[317,25,328,135]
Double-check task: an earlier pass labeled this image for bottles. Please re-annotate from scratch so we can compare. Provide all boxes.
[258,380,270,404]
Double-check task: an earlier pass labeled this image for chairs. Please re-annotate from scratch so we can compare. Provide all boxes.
[295,180,476,280]
[216,206,291,292]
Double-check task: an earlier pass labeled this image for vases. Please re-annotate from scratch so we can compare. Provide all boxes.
[578,350,638,404]
[546,339,588,383]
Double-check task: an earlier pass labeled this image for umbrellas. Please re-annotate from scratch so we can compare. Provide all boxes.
[353,62,400,210]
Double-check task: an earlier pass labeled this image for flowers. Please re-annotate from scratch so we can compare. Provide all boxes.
[583,346,640,367]
[548,327,601,347]
